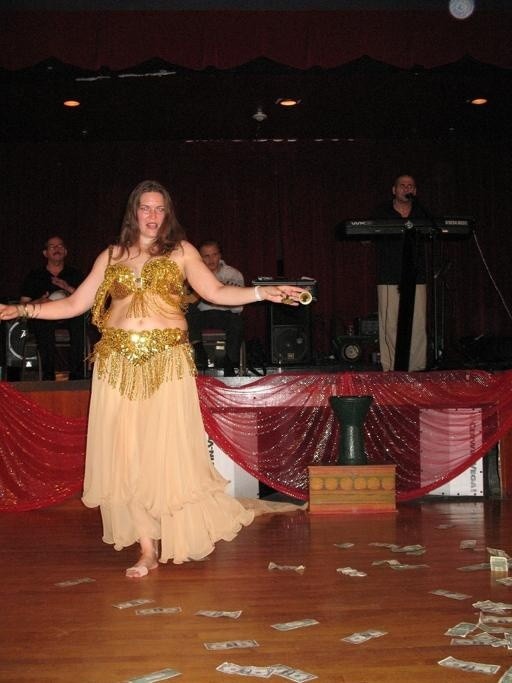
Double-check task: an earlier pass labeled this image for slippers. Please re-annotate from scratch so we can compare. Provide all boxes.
[124,563,159,578]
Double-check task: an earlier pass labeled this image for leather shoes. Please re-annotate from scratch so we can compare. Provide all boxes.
[193,342,208,369]
[224,360,235,377]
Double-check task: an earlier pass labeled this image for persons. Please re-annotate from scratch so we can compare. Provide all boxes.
[374,173,429,373]
[185,239,245,377]
[20,236,84,380]
[1,179,308,578]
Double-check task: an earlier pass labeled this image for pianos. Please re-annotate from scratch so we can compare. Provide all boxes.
[334,218,475,241]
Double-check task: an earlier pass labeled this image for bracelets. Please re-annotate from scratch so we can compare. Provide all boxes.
[16,302,41,319]
[255,286,262,301]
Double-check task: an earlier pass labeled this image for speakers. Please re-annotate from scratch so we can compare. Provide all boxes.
[266,303,310,366]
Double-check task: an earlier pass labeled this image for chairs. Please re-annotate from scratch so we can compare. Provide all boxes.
[200,304,248,374]
[54,327,91,379]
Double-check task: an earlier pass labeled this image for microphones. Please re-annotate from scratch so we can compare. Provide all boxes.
[405,193,416,201]
